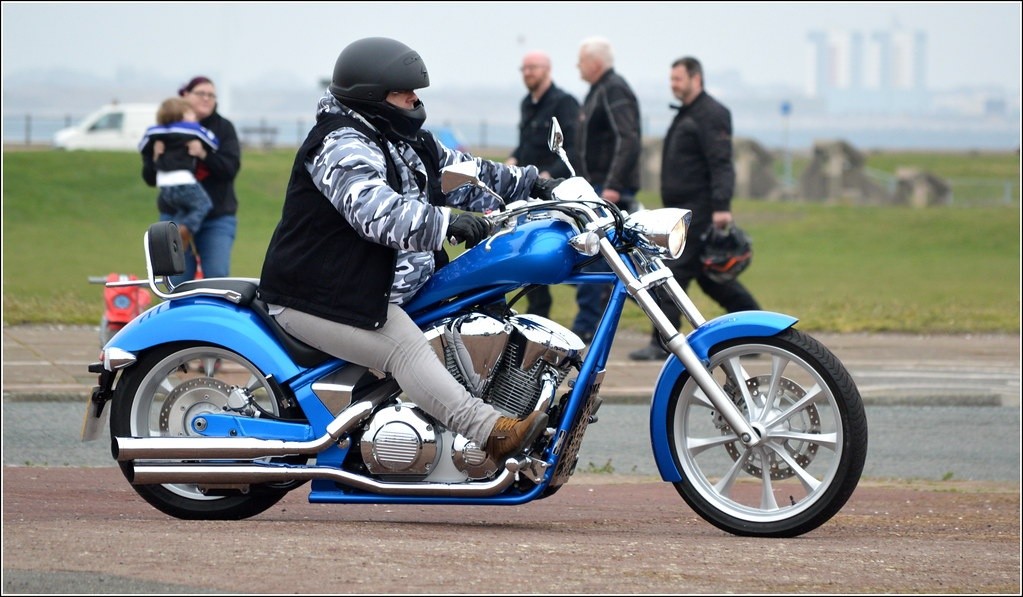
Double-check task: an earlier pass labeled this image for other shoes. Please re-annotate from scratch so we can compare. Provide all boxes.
[177,226,189,252]
[629,344,671,360]
[571,331,594,343]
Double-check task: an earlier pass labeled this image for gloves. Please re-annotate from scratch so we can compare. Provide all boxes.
[446,212,489,249]
[537,178,565,201]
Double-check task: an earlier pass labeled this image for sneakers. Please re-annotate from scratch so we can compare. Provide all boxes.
[484,410,548,470]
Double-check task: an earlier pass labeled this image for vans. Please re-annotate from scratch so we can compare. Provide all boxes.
[55,103,159,153]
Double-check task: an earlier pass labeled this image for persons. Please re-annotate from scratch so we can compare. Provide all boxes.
[138,98,218,255]
[255,36,567,474]
[629,57,761,364]
[572,39,641,344]
[502,52,582,317]
[142,77,240,378]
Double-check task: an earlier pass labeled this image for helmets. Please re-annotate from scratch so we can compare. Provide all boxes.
[329,37,429,142]
[702,224,751,282]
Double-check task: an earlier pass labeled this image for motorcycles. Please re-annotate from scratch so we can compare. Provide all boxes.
[79,115,868,538]
[86,273,152,349]
